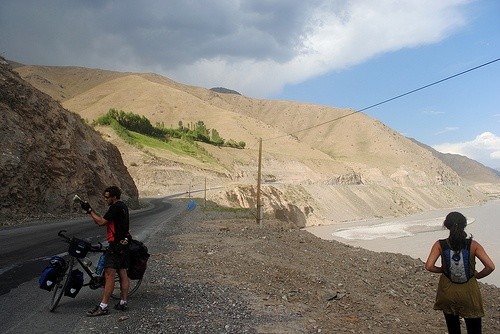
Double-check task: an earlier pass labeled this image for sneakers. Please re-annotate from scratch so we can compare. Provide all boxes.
[114,301,128,311]
[86,305,109,317]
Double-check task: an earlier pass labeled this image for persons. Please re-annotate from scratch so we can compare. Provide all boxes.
[80,186,132,317]
[425,212,495,334]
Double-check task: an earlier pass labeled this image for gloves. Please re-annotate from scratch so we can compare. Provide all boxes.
[81,202,92,214]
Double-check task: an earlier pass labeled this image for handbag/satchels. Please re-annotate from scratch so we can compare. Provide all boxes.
[38,266,58,292]
[64,268,84,298]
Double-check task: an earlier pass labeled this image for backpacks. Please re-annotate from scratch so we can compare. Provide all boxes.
[438,237,472,284]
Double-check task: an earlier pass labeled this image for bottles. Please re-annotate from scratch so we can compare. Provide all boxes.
[83,257,96,273]
[95,253,105,275]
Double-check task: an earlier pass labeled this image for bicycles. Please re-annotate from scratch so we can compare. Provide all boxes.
[48,229,144,313]
